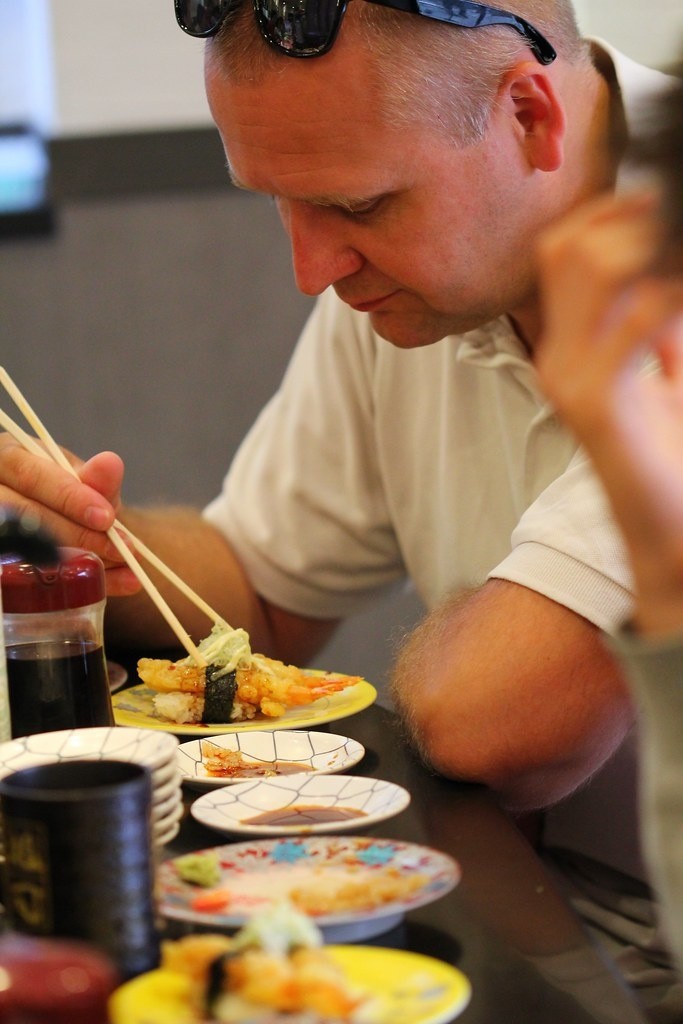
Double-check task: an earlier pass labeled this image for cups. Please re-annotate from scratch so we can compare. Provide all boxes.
[0,760,162,986]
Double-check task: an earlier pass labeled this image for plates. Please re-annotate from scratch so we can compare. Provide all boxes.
[154,837,460,941]
[110,946,471,1024]
[106,662,127,691]
[110,670,377,735]
[0,727,184,849]
[190,776,410,834]
[177,731,365,786]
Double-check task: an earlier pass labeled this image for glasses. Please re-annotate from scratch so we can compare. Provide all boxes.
[175,0,558,66]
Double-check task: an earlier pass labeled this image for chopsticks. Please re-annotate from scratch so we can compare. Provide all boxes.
[0,365,234,670]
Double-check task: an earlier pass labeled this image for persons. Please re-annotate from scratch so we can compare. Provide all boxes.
[0,1,683,1024]
[535,79,682,981]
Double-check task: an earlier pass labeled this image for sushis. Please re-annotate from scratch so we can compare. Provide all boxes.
[162,907,324,972]
[192,953,382,1024]
[139,663,256,719]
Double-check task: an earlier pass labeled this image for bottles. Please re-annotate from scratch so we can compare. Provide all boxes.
[0,548,114,740]
